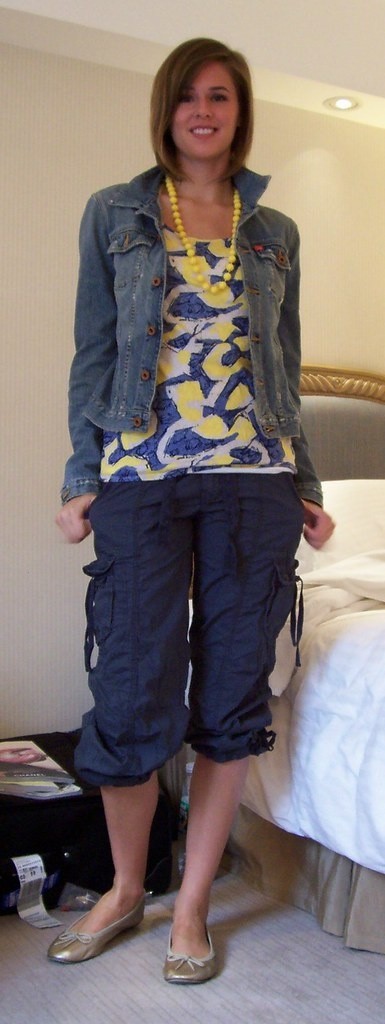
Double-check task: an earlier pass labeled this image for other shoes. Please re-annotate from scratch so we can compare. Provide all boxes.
[162,922,217,983]
[48,890,146,963]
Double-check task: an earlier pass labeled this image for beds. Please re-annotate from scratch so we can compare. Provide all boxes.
[158,367,385,955]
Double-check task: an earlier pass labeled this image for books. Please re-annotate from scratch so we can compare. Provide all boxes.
[0,741,83,801]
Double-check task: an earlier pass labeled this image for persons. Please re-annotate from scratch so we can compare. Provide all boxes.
[49,37,334,986]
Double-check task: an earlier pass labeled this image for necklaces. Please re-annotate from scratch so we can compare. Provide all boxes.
[164,172,241,295]
